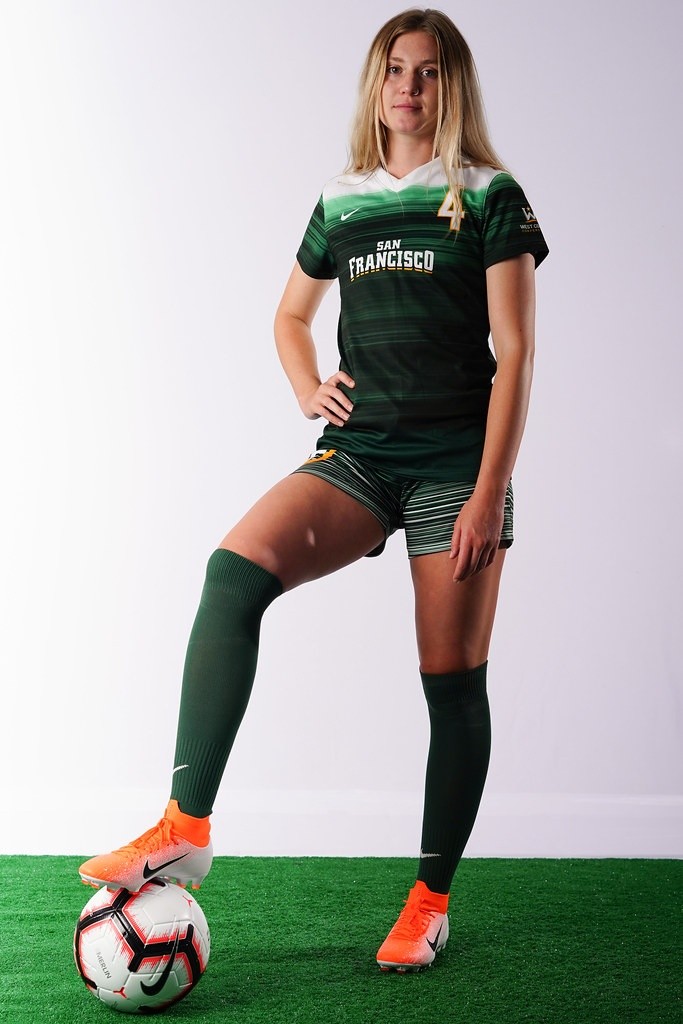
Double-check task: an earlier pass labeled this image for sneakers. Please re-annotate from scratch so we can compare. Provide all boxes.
[375,881,449,973]
[78,800,212,894]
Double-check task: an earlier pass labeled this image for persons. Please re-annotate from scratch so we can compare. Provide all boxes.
[76,7,548,968]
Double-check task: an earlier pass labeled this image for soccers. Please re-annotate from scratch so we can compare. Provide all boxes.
[72,880,211,1014]
